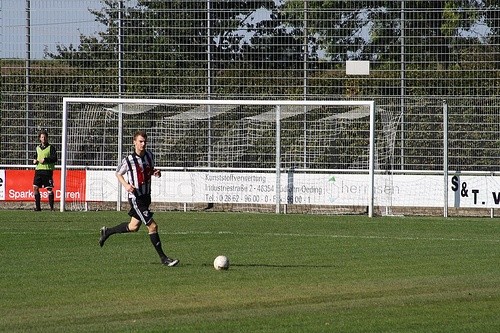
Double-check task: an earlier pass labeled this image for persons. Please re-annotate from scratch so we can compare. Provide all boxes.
[99,132,180,267]
[32,129,58,212]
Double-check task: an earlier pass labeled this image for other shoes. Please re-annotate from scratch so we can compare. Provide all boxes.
[162,257,179,266]
[99,226,108,247]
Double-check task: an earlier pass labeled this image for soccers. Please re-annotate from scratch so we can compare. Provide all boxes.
[213,254,232,271]
